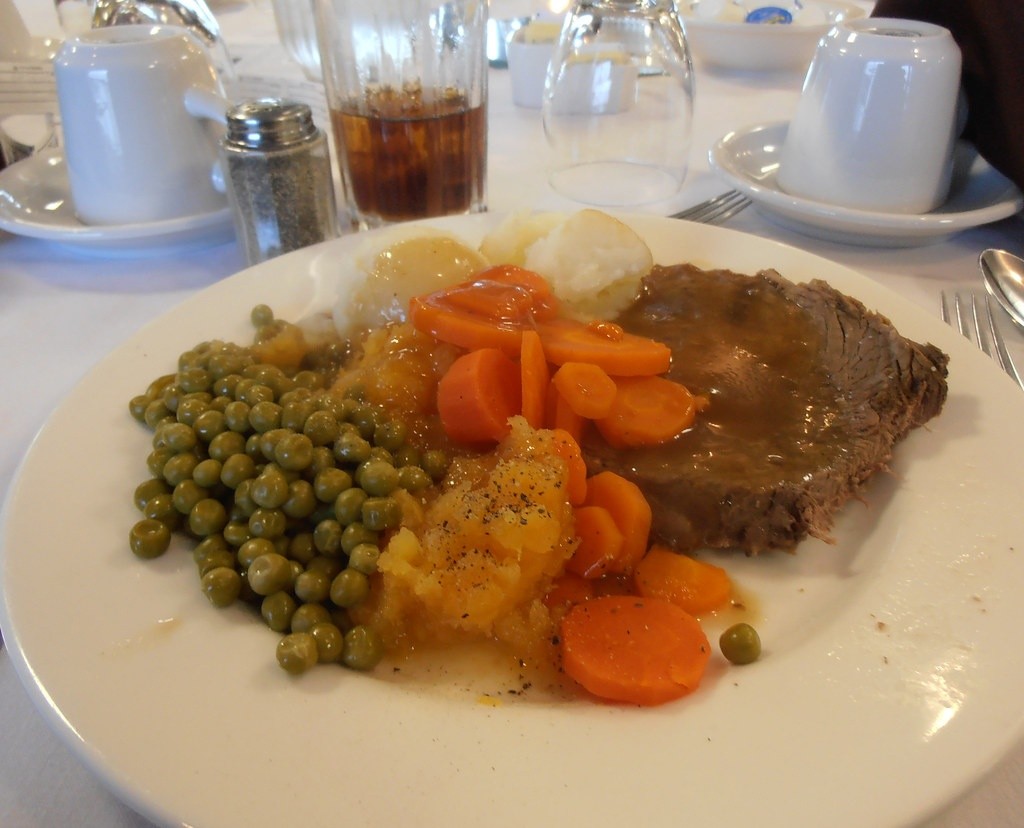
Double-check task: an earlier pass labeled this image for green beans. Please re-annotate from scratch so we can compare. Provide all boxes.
[125,304,761,670]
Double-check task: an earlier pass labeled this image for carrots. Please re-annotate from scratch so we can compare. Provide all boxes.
[403,264,732,706]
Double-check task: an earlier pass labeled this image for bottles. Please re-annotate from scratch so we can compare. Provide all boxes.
[220,99,339,269]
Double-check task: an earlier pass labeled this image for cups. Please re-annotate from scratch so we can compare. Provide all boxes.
[58,24,244,229]
[311,0,490,235]
[539,1,694,208]
[776,16,967,217]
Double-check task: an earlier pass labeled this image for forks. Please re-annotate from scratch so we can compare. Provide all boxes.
[671,188,754,228]
[937,291,1024,397]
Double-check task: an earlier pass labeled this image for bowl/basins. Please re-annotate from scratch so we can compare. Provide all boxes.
[677,0,864,74]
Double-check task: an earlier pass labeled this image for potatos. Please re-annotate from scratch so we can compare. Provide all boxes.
[358,205,654,329]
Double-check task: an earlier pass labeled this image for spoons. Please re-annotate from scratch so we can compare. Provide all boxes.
[978,247,1024,333]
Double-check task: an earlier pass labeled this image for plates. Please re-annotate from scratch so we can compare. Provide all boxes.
[1,202,1023,828]
[705,114,1024,247]
[0,145,245,244]
